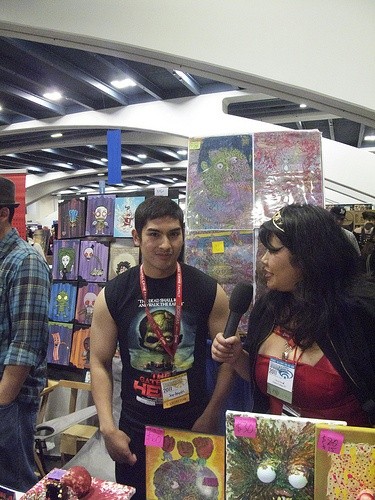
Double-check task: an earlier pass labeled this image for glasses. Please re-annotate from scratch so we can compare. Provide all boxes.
[272,211,286,233]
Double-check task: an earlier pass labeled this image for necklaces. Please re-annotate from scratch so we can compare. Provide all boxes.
[283,341,291,358]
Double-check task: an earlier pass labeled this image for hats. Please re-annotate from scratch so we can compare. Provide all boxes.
[0,177,20,208]
[331,206,346,220]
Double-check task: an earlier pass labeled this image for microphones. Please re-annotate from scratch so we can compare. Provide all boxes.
[216,280,253,366]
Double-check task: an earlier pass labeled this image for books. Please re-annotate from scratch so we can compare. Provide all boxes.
[20,467,136,500]
[225,409,347,500]
[0,486,15,500]
[314,423,375,500]
[145,423,225,500]
[47,196,147,369]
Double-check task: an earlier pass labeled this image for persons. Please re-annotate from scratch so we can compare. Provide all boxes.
[90,195,241,500]
[329,205,361,257]
[211,202,375,428]
[0,177,53,493]
